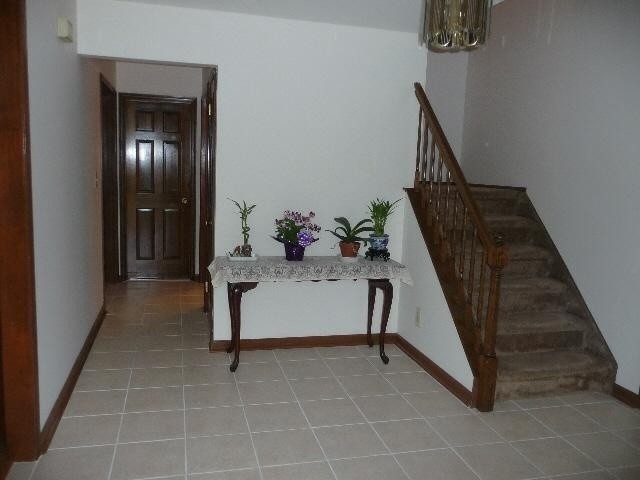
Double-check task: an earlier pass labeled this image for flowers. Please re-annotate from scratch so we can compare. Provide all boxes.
[268,210,321,248]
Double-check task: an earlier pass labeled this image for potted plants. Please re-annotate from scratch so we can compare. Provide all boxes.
[325,217,377,257]
[365,198,404,262]
[226,197,259,262]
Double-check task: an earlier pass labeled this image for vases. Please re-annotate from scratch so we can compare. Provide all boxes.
[284,244,305,261]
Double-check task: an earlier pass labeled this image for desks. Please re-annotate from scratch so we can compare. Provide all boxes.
[216,256,408,373]
